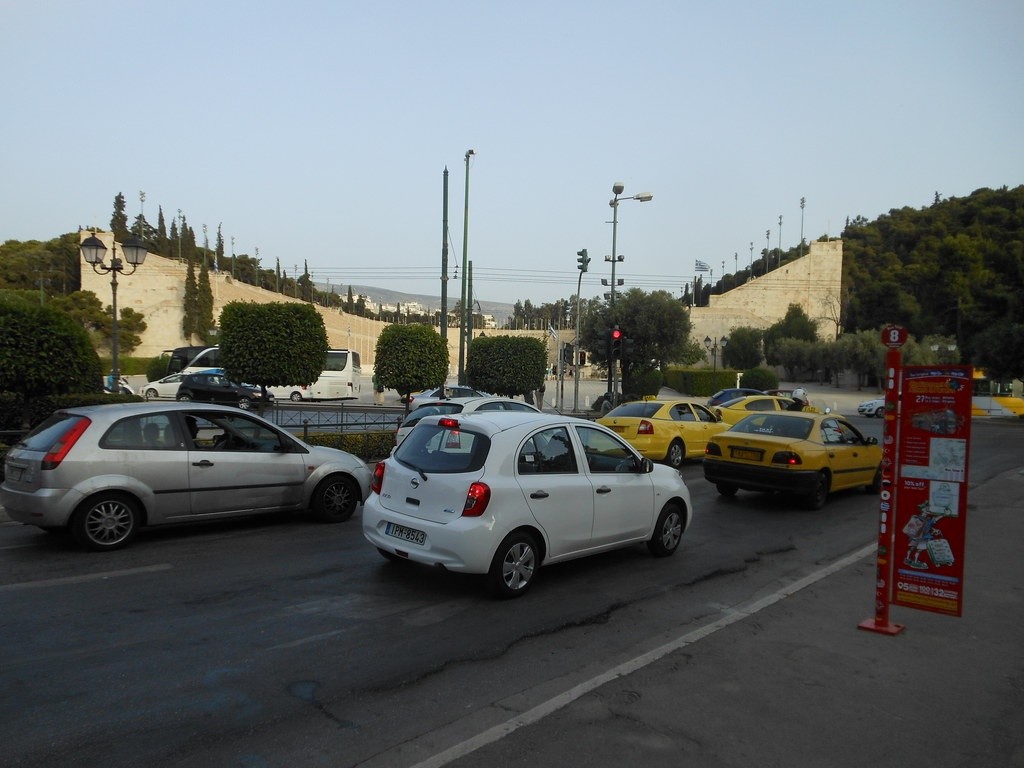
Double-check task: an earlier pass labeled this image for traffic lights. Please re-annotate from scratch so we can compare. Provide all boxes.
[580,352,586,365]
[577,250,589,272]
[612,329,622,358]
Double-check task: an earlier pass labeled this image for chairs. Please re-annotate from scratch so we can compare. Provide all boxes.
[560,455,599,472]
[519,463,537,474]
[164,424,183,447]
[142,424,164,447]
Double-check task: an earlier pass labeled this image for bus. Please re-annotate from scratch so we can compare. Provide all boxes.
[159,344,362,403]
[970,366,1024,421]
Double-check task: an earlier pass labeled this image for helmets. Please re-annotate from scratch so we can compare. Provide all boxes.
[792,386,809,402]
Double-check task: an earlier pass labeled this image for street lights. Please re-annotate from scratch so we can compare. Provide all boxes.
[203,224,208,269]
[722,261,725,292]
[750,242,754,280]
[800,196,805,257]
[177,208,184,261]
[766,229,770,273]
[310,271,314,299]
[138,190,145,241]
[80,232,148,395]
[735,252,738,288]
[326,277,329,308]
[710,269,713,294]
[294,264,298,298]
[231,235,235,278]
[256,246,258,284]
[681,287,684,304]
[778,215,782,268]
[602,184,654,407]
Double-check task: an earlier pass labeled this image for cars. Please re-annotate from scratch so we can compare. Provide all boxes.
[594,388,885,509]
[141,371,276,411]
[858,392,904,419]
[363,385,693,599]
[0,401,374,552]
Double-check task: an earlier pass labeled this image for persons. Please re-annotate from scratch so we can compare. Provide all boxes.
[787,389,816,412]
[535,382,545,412]
[185,416,213,449]
[371,369,383,406]
